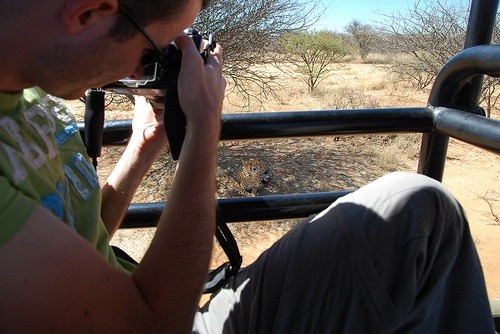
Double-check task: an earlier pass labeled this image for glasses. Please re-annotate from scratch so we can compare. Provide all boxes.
[118,8,170,63]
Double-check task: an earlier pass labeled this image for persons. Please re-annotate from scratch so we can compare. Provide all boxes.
[1,0,497,334]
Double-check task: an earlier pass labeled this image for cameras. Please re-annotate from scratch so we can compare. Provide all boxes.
[82,25,204,158]
